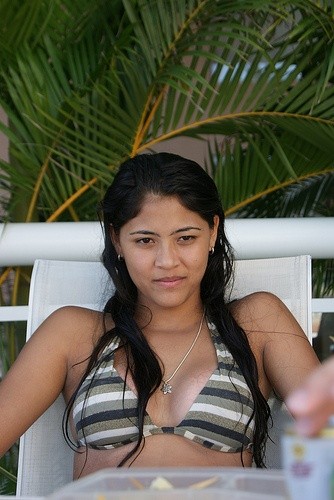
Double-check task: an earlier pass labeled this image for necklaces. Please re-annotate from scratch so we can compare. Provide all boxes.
[133,303,207,394]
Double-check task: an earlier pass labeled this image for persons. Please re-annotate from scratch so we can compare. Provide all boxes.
[0,149,334,500]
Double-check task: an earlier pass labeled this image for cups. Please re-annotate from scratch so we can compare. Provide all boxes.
[280,433,332,500]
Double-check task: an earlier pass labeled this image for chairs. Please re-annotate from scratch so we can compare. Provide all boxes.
[1,219,331,500]
